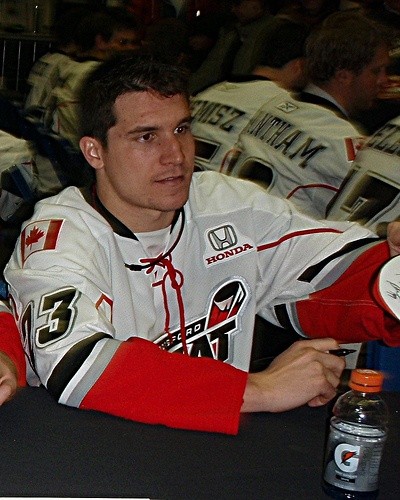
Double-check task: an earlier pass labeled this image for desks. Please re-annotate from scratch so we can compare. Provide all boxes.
[0,385,400,500]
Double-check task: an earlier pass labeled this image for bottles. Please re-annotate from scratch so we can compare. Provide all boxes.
[321,369,390,500]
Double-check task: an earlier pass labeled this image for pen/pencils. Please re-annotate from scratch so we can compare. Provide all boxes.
[325,348,358,357]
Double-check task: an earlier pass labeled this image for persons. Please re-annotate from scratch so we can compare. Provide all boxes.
[323,114,400,222]
[221,10,400,221]
[186,18,306,174]
[0,48,400,414]
[0,0,400,181]
[0,298,27,411]
